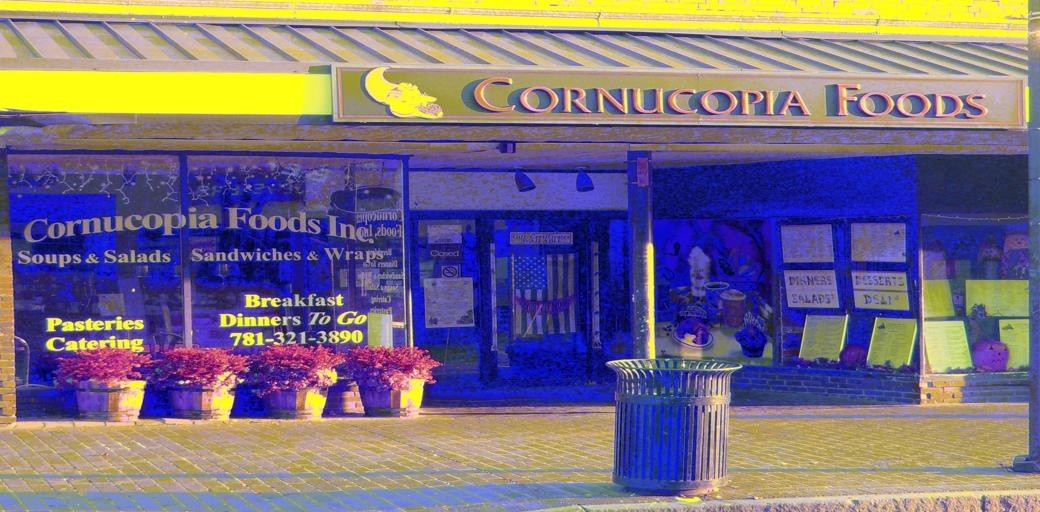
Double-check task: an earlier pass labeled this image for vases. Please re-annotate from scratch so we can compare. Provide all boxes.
[266,380,328,417]
[359,375,428,415]
[75,377,147,425]
[163,376,238,419]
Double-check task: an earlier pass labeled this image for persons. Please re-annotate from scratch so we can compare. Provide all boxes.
[460,224,478,286]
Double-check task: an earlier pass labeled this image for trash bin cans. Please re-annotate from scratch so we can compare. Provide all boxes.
[604,357,742,495]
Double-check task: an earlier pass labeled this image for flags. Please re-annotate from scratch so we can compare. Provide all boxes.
[506,250,579,336]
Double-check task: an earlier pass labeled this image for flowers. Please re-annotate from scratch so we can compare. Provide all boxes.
[350,343,443,396]
[54,347,154,399]
[151,341,250,382]
[245,344,348,397]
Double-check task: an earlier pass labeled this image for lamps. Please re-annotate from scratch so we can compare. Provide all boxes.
[499,142,516,154]
[515,172,536,191]
[576,171,595,191]
